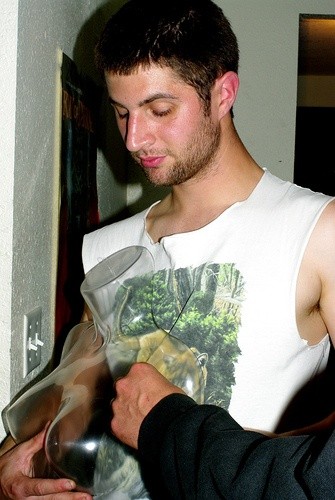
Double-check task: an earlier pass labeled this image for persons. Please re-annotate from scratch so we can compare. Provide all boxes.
[108,361,335,500]
[1,1,335,499]
